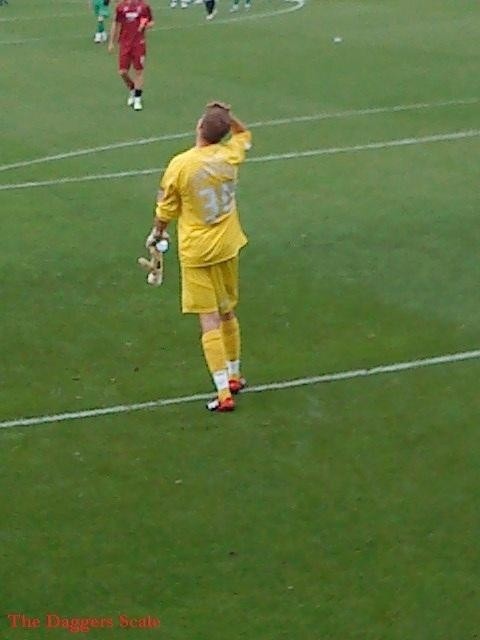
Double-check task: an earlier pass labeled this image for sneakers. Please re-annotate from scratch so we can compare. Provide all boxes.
[228,3,252,14]
[228,375,248,394]
[127,88,142,111]
[207,10,217,21]
[171,1,187,9]
[95,30,107,42]
[206,396,236,412]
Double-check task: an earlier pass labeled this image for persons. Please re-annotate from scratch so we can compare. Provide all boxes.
[169,0,251,21]
[89,0,112,43]
[107,0,154,110]
[146,102,252,411]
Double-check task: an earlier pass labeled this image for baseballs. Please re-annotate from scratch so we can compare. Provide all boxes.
[334,37,341,43]
[156,239,167,251]
